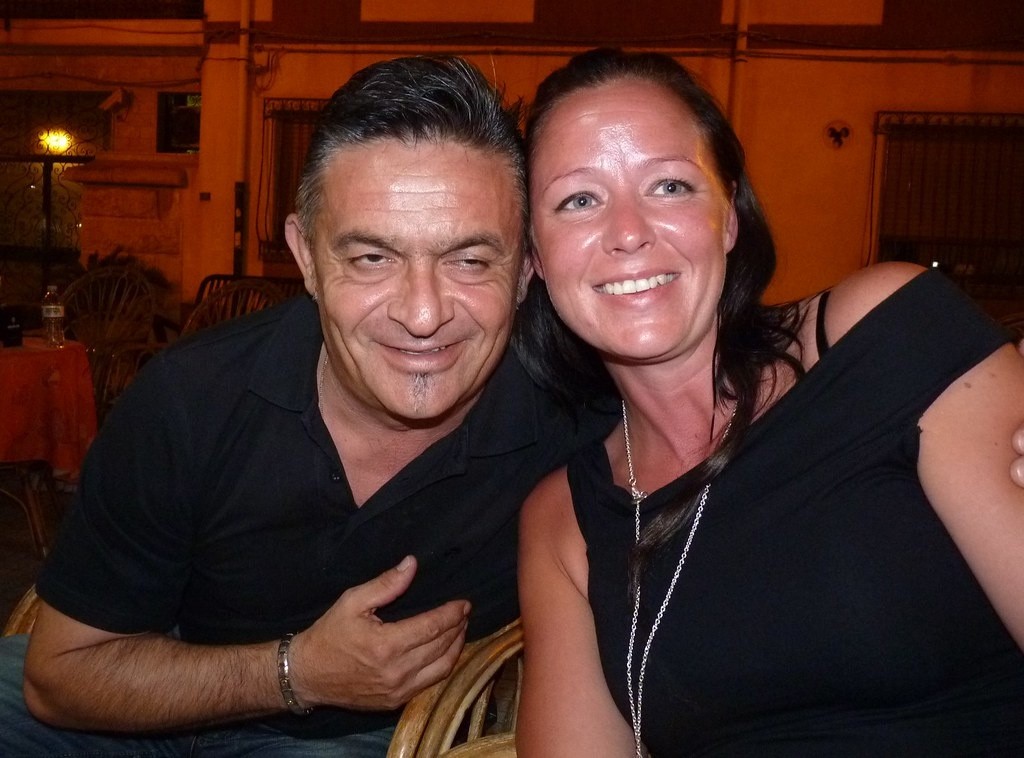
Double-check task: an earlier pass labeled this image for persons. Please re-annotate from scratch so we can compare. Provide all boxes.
[515,47,1024,758]
[0,53,1024,758]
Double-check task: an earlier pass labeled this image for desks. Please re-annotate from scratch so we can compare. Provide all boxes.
[0,337,99,567]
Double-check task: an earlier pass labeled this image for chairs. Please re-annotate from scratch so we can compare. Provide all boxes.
[60,265,156,425]
[180,279,285,334]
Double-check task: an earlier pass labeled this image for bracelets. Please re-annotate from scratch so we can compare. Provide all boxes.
[277,633,313,717]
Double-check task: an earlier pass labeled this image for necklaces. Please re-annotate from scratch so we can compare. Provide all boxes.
[319,354,330,414]
[622,399,737,758]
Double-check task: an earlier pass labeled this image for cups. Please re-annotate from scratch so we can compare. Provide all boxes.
[3,319,22,347]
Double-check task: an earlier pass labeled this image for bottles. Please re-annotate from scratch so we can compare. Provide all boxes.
[41,285,66,348]
